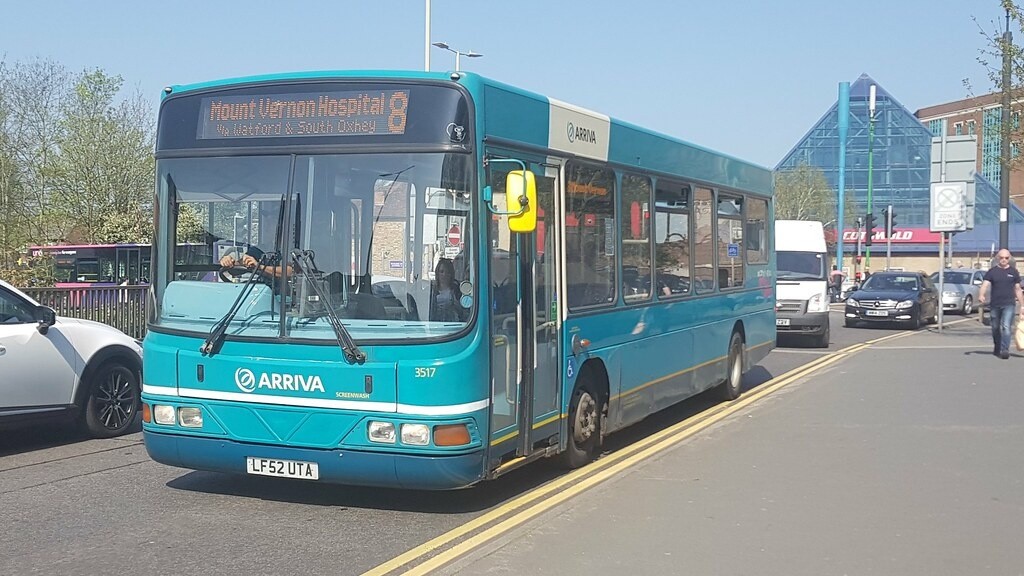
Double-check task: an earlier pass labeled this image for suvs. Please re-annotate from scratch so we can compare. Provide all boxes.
[0,279,145,439]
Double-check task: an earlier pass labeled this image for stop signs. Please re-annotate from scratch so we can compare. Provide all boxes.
[448,226,460,247]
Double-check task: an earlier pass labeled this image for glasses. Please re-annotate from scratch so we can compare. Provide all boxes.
[1000,257,1009,260]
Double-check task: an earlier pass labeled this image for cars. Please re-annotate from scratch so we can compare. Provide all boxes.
[930,268,991,315]
[844,271,938,330]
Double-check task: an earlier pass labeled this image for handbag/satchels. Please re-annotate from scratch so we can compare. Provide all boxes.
[1015,320,1024,350]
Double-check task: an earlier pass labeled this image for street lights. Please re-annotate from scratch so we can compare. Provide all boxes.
[233,213,244,247]
[433,41,484,71]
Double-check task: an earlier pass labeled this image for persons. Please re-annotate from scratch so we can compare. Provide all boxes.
[217,203,293,293]
[542,232,671,307]
[827,265,871,303]
[428,258,465,322]
[978,249,1024,359]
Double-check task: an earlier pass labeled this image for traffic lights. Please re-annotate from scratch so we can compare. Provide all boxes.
[865,213,877,246]
[891,210,898,237]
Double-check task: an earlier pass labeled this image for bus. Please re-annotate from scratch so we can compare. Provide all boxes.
[17,239,248,309]
[141,69,778,491]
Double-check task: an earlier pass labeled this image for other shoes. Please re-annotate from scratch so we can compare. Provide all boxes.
[993,350,1000,356]
[1002,352,1009,359]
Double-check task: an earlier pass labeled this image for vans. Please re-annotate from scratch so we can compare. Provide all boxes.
[773,219,841,349]
